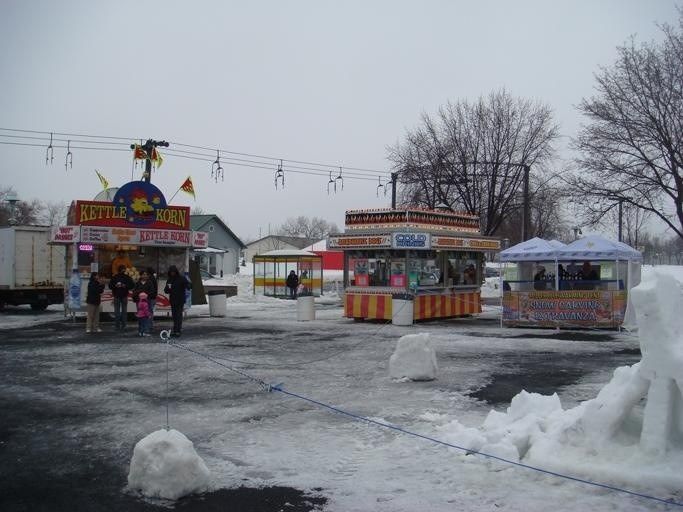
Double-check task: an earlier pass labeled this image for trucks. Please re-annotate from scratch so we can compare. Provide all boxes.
[0,224,66,310]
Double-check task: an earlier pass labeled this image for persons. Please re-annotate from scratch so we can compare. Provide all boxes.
[85,271,106,333]
[300,270,309,294]
[135,292,152,337]
[164,264,193,338]
[534,265,547,291]
[133,269,156,333]
[442,260,455,279]
[108,263,135,331]
[551,263,574,289]
[464,263,476,284]
[111,249,132,275]
[147,264,158,311]
[575,261,601,290]
[286,270,299,300]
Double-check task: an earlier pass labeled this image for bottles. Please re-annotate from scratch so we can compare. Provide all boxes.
[68,269,81,309]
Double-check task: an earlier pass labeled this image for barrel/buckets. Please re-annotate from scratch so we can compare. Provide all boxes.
[391,295,414,325]
[296,293,316,321]
[207,289,227,317]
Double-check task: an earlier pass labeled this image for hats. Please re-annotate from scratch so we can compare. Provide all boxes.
[138,292,148,299]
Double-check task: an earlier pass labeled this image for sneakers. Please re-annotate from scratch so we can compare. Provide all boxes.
[138,332,150,337]
[86,328,91,333]
[96,329,102,333]
[169,331,180,336]
